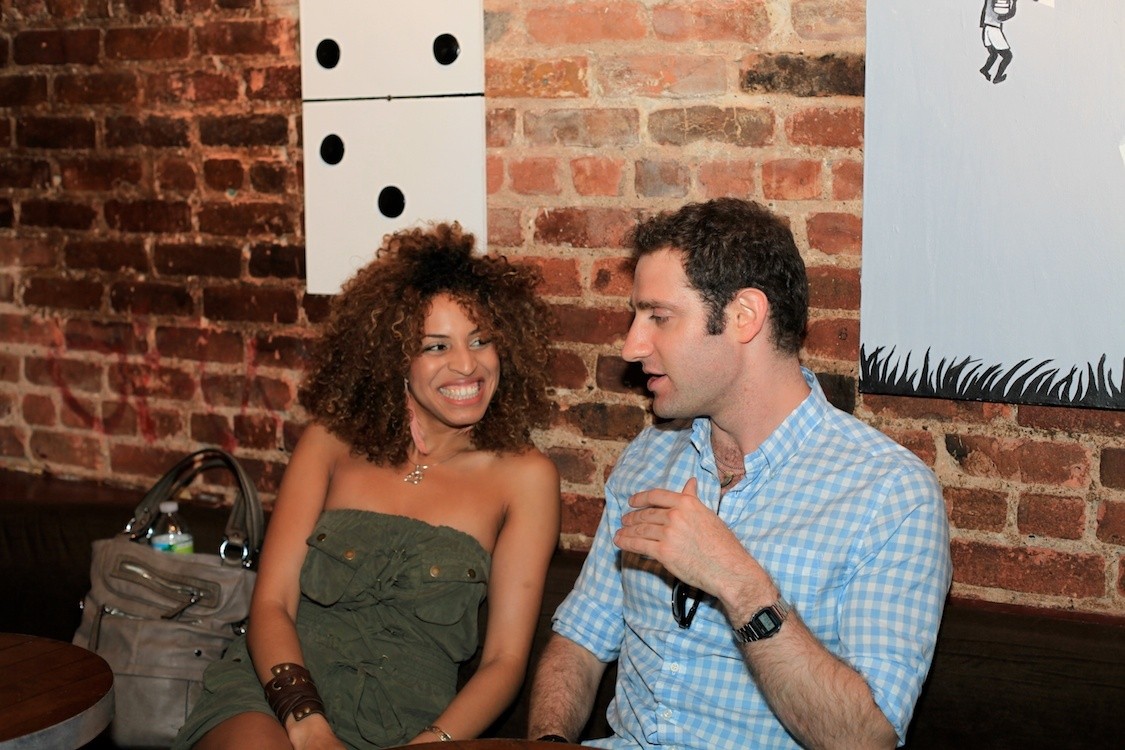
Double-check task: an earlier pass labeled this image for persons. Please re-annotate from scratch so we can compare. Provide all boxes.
[526,193,954,749]
[169,218,560,750]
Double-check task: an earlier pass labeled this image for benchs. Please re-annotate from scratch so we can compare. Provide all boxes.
[0,466,1125,750]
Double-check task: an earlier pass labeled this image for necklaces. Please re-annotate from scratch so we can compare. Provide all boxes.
[403,448,468,485]
[714,451,746,489]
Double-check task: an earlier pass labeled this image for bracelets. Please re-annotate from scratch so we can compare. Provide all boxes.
[424,724,451,744]
[536,734,568,744]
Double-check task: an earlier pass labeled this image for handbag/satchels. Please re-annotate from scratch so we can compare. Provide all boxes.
[70,447,265,747]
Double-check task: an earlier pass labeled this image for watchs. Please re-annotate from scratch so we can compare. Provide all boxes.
[731,596,790,645]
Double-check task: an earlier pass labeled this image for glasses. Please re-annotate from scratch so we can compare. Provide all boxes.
[672,581,705,628]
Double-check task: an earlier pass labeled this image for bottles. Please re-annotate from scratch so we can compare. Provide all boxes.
[149,501,193,553]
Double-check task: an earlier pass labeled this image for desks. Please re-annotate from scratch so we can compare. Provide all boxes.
[1,631,118,750]
